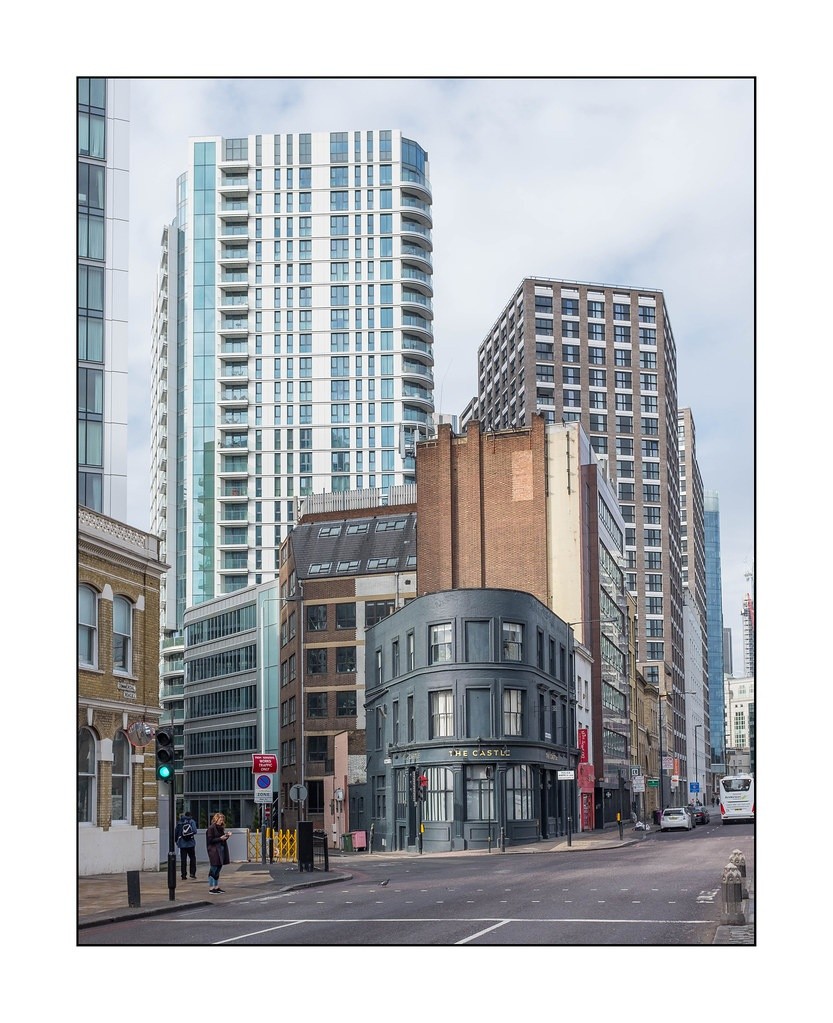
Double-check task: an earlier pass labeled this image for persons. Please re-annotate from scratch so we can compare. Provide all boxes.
[711,796,715,807]
[176,811,197,880]
[206,813,232,894]
[716,797,720,804]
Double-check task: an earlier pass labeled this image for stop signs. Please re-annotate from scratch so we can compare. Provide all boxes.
[264,807,271,818]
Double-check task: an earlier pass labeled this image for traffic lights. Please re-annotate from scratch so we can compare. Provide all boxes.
[154,727,176,783]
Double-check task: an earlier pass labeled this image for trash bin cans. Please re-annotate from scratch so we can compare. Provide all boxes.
[341,833,352,852]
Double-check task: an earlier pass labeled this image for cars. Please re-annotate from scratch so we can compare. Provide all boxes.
[691,806,710,825]
[660,805,694,832]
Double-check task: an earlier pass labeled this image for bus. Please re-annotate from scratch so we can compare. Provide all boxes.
[718,773,755,825]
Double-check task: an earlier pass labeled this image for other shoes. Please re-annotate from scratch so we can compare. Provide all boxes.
[182,877,187,880]
[190,875,197,879]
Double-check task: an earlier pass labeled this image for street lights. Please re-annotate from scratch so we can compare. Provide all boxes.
[566,616,619,846]
[261,595,305,865]
[660,690,697,814]
[724,734,730,776]
[695,724,703,807]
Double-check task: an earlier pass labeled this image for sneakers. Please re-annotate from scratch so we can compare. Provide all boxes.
[216,887,226,892]
[209,888,221,895]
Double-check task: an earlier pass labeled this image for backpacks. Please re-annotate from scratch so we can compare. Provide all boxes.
[181,819,195,842]
